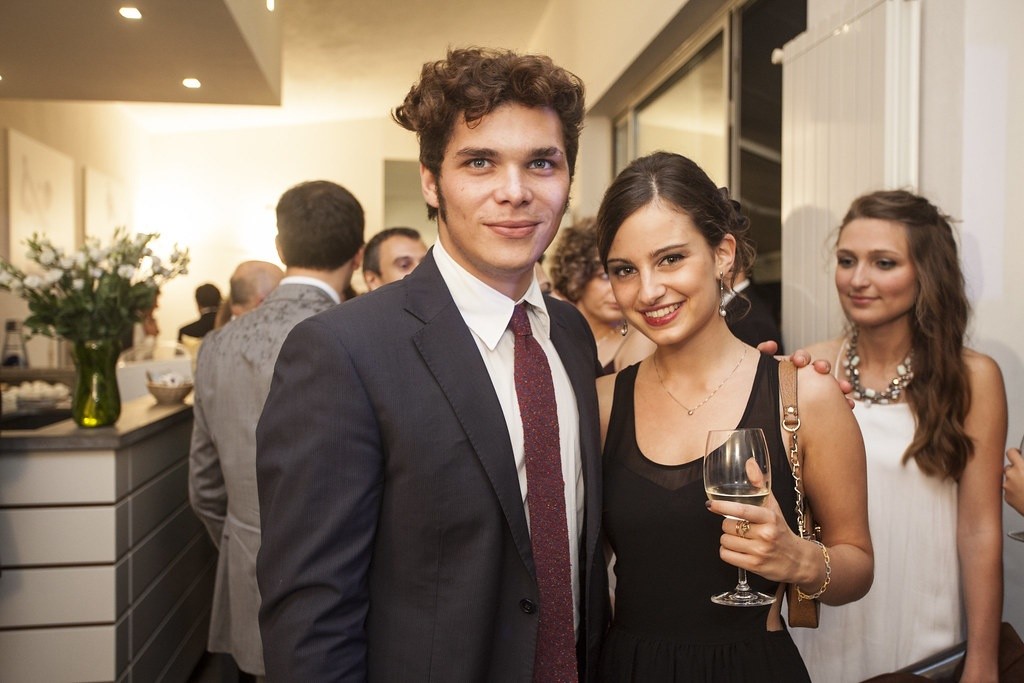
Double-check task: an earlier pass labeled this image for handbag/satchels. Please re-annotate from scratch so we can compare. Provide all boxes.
[766,361,821,635]
[858,621,1024,683]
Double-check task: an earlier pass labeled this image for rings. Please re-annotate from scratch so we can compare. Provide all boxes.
[735,520,751,537]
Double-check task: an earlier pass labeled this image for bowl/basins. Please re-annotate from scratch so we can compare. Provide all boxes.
[147,382,193,404]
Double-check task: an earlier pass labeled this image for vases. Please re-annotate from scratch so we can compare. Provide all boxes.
[64,336,124,428]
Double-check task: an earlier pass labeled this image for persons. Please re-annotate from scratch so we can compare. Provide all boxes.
[175,211,783,516]
[593,151,875,683]
[189,181,368,683]
[258,45,857,683]
[1002,447,1024,516]
[792,188,1007,683]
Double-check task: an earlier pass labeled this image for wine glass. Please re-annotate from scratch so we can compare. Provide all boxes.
[703,428,778,605]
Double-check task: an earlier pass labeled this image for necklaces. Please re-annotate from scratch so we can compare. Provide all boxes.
[653,341,748,416]
[846,328,918,408]
[590,322,625,347]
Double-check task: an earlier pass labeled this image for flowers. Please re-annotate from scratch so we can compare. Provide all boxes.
[0,226,190,422]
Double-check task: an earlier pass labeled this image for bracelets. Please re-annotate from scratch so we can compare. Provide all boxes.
[793,538,832,604]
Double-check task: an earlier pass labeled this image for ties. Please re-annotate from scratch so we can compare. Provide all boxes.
[506,301,579,683]
